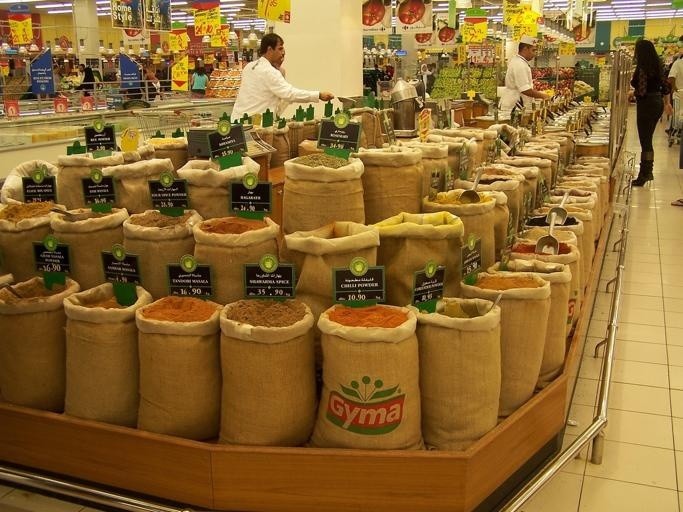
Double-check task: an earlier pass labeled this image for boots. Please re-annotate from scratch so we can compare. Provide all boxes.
[632,150,655,186]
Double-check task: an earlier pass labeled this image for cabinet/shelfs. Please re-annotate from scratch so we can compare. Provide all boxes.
[426,63,596,109]
[204,67,244,99]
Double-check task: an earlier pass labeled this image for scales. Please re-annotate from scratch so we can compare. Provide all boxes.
[461,91,495,107]
[188,123,278,158]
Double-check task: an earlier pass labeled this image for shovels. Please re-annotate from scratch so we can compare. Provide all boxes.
[458,171,482,204]
[50,208,88,222]
[545,192,569,226]
[535,212,559,254]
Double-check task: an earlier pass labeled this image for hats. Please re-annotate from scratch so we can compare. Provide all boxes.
[519,35,540,46]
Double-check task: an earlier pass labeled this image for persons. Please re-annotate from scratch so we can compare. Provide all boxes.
[230,33,334,125]
[74,63,95,96]
[272,47,292,118]
[416,64,435,95]
[497,37,549,113]
[368,64,384,97]
[628,35,683,206]
[190,67,212,97]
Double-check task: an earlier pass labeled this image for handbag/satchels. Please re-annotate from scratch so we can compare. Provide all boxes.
[633,64,648,98]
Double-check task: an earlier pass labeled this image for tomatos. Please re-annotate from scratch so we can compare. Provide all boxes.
[439,26,455,43]
[398,0,425,25]
[415,34,432,44]
[574,24,591,41]
[362,1,385,26]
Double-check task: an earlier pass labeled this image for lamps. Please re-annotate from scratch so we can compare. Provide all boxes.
[362,43,403,60]
[538,15,574,40]
[0,3,275,63]
